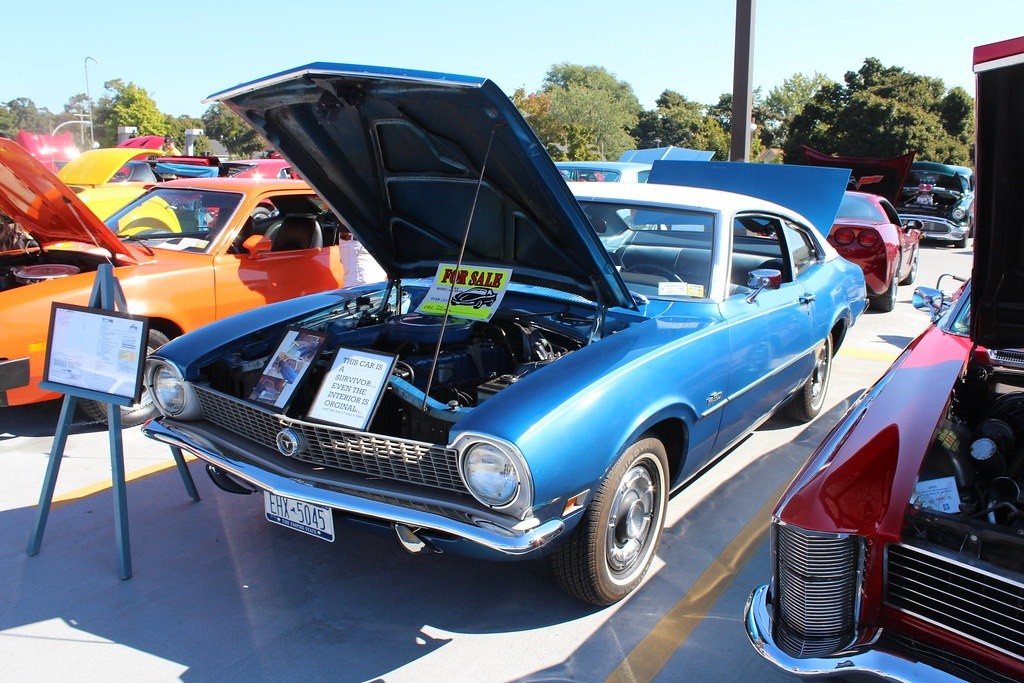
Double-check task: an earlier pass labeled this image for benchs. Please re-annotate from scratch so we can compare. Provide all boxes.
[615,245,783,296]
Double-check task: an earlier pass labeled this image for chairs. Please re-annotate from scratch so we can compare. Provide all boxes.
[264,198,324,252]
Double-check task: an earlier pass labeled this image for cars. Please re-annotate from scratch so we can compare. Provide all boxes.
[137,59,871,611]
[0,131,354,431]
[554,143,718,185]
[739,36,1024,683]
[893,161,975,248]
[794,143,925,312]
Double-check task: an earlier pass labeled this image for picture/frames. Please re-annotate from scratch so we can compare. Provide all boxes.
[302,346,400,432]
[242,325,332,415]
[42,302,149,404]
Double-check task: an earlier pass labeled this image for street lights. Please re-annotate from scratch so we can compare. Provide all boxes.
[85,53,104,152]
[52,118,92,138]
